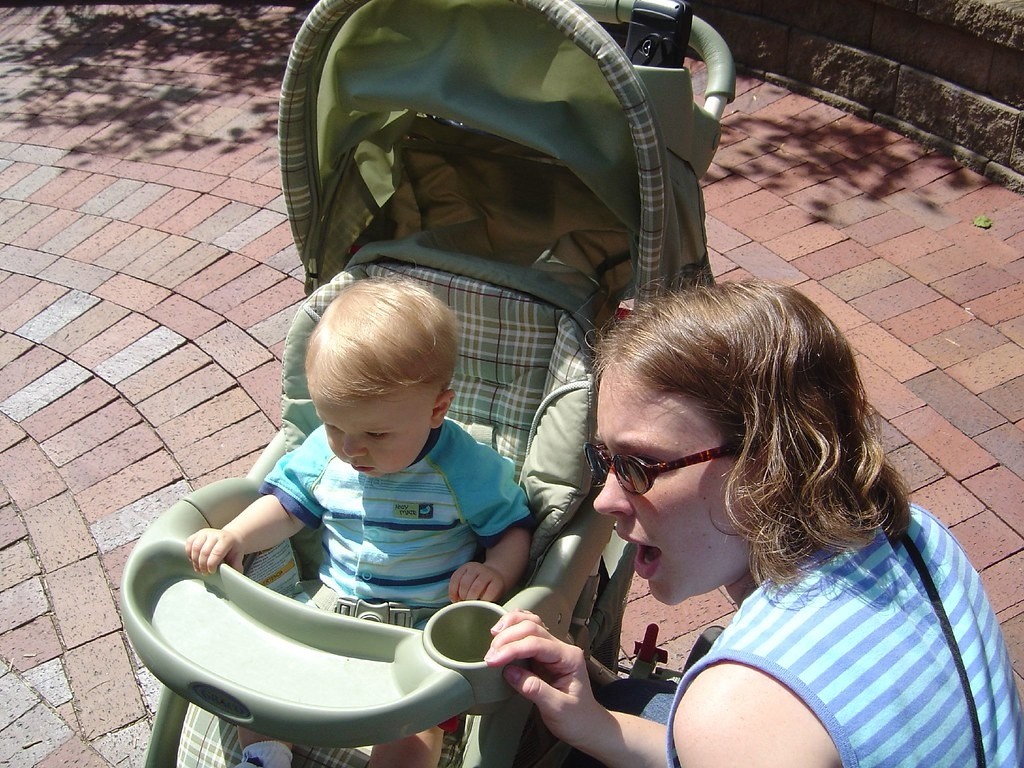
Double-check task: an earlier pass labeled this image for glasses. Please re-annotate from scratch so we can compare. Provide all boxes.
[583,442,760,496]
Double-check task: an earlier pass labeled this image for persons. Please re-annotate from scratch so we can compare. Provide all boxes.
[185,277,531,768]
[483,278,1024,768]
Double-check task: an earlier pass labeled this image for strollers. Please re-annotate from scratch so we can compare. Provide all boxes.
[120,1,737,768]
